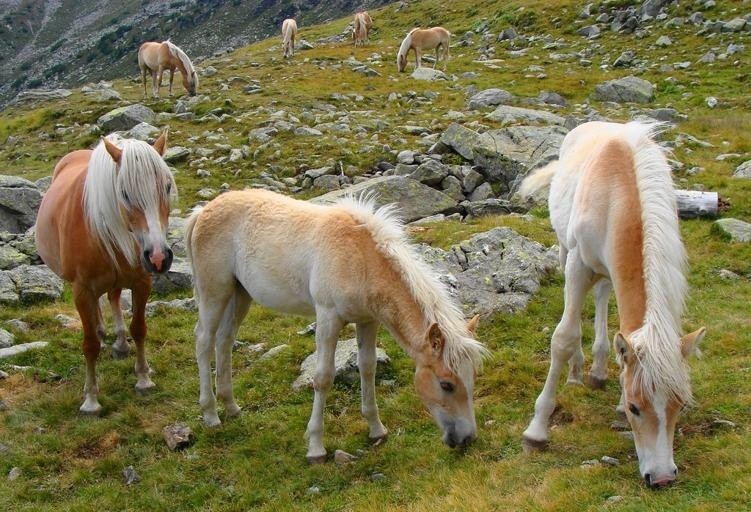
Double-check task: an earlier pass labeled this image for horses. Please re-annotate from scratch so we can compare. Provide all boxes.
[182,186,495,465]
[521,114,706,492]
[34,125,178,416]
[348,12,373,49]
[281,18,297,58]
[395,27,452,74]
[137,39,200,100]
